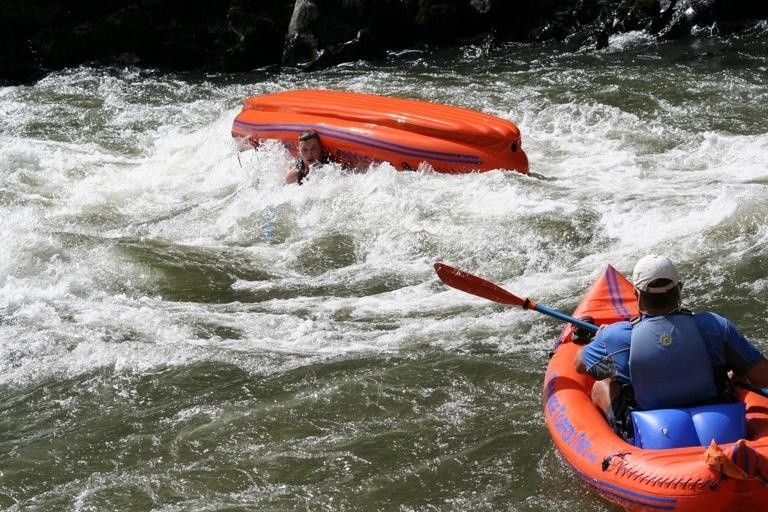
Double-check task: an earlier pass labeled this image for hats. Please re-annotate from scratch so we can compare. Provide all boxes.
[631,253,679,294]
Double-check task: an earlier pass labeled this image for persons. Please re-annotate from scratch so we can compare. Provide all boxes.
[286,130,333,187]
[574,254,766,441]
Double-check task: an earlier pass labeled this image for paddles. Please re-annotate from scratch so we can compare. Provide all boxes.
[433,262,767,401]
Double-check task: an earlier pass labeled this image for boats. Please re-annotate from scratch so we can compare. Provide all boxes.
[543,264,768,512]
[231,90,530,174]
